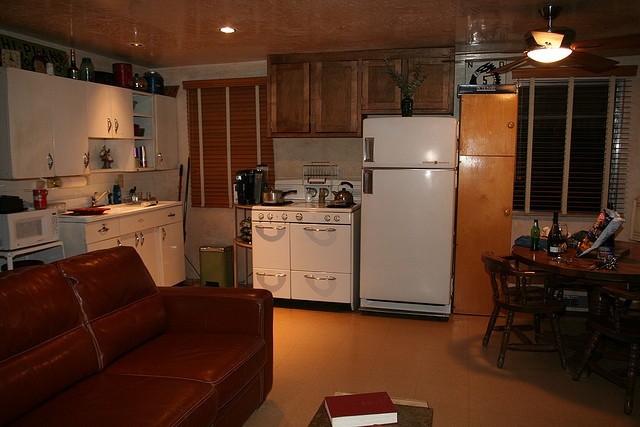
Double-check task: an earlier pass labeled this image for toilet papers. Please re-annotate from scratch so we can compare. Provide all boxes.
[58,176,86,188]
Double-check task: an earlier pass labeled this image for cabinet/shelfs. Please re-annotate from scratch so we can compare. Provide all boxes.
[158,202,186,287]
[50,76,89,175]
[362,46,456,114]
[58,214,120,258]
[120,207,160,289]
[232,203,256,289]
[152,92,180,171]
[452,92,519,316]
[88,82,136,174]
[265,50,362,138]
[133,90,156,170]
[0,67,55,181]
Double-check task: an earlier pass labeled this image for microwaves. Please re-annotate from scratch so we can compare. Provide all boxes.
[0,206,61,250]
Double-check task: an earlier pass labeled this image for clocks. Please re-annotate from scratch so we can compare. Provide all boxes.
[2,49,21,68]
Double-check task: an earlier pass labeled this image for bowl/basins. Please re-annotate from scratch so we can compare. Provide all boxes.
[123,197,136,205]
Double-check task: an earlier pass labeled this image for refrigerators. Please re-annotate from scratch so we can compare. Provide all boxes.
[358,115,460,320]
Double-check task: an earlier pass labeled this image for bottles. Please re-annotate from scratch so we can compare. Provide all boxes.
[530,218,541,251]
[546,211,560,257]
[80,57,95,81]
[319,186,325,202]
[113,179,122,204]
[67,48,81,79]
[33,188,48,210]
[305,187,312,202]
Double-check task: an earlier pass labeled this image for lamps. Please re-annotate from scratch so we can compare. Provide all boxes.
[527,47,573,64]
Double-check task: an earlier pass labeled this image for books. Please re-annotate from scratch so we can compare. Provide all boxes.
[323,390,400,426]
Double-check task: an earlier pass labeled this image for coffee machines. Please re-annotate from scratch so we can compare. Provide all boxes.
[235,168,257,205]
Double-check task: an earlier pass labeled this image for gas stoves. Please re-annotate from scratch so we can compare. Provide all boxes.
[251,198,361,213]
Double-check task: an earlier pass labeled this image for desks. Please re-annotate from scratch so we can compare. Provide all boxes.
[0,240,65,270]
[512,235,639,356]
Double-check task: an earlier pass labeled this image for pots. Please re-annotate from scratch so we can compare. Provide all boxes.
[262,186,297,203]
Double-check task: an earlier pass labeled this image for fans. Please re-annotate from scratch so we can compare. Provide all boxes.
[491,5,620,73]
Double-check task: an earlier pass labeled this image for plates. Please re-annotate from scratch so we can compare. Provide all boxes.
[67,207,111,214]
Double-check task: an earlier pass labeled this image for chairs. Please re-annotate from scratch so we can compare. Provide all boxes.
[570,283,640,417]
[479,249,569,370]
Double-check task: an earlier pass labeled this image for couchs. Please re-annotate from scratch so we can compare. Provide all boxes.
[0,244,274,426]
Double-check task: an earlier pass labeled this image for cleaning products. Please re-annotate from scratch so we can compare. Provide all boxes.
[113,185,122,205]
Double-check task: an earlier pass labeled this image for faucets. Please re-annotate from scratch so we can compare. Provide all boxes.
[90,189,110,206]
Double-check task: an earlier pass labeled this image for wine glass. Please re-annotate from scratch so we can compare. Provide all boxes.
[558,222,568,244]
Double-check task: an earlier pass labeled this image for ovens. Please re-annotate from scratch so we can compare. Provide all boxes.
[251,209,359,311]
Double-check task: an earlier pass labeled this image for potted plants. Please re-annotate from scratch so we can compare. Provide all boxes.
[382,56,428,116]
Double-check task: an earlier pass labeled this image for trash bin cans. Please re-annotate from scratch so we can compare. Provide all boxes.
[199,245,234,288]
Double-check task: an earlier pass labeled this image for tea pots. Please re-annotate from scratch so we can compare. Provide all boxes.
[331,181,354,203]
